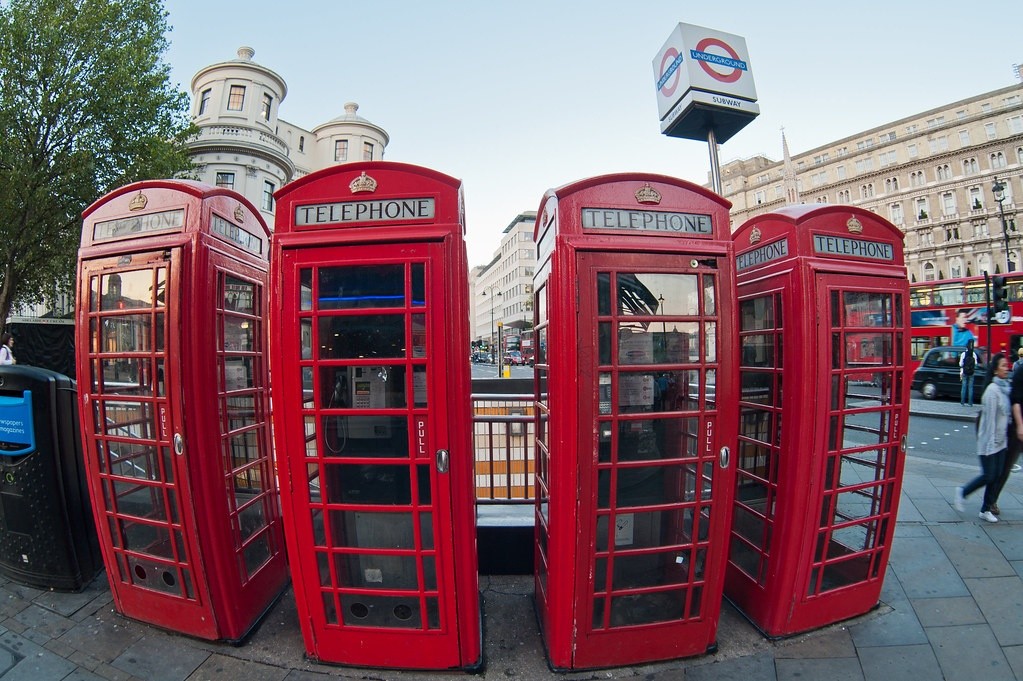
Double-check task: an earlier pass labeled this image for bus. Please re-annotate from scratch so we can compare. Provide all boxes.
[844,269,1023,384]
[520,330,535,365]
[503,335,520,352]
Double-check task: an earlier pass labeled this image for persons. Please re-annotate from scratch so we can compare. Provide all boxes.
[989,361,1023,515]
[127,346,139,383]
[953,353,1012,522]
[959,342,978,406]
[0,332,16,366]
[1010,347,1023,376]
[301,341,313,390]
[950,310,979,361]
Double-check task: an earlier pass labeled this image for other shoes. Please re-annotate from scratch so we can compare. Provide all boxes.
[979,511,998,523]
[990,503,1000,515]
[954,487,965,512]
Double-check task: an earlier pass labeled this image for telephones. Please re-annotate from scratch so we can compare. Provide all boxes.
[329,351,399,443]
[599,365,658,444]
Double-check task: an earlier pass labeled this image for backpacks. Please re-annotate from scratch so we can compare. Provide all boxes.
[963,338,975,374]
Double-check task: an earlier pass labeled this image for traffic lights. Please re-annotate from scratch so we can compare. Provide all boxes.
[477,340,482,348]
[992,275,1008,313]
[472,341,476,349]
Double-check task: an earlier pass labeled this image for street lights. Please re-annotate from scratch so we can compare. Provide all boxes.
[659,294,666,358]
[482,284,503,365]
[992,175,1011,272]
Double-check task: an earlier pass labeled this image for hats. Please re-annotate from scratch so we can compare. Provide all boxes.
[1017,348,1023,354]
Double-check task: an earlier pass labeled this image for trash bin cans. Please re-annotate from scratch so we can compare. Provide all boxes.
[0,363,104,596]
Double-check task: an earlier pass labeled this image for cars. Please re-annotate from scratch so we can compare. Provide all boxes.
[910,346,1014,403]
[474,350,525,367]
